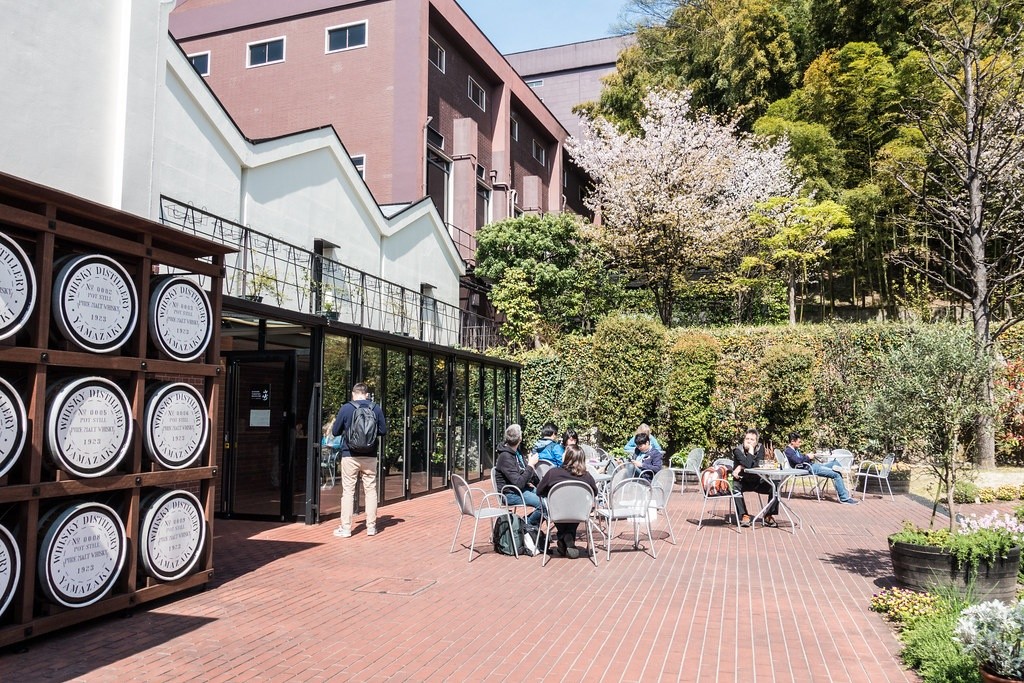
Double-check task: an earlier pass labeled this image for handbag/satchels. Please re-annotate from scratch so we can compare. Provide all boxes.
[522,526,554,557]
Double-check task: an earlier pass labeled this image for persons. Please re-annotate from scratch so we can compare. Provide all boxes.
[732,429,779,528]
[322,414,336,446]
[497,423,663,559]
[332,383,387,538]
[786,432,860,505]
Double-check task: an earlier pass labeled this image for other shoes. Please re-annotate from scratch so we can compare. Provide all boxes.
[564,533,580,559]
[557,541,566,556]
[590,512,594,516]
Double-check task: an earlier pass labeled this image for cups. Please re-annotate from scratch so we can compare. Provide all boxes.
[823,448,829,454]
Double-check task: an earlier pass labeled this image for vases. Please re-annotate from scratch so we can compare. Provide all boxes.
[887,532,1021,607]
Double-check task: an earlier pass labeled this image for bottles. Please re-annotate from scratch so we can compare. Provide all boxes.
[530,448,536,457]
[727,473,734,492]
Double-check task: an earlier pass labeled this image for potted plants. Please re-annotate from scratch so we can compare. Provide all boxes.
[859,462,912,494]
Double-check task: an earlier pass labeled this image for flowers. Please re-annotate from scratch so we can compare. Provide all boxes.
[887,508,1024,587]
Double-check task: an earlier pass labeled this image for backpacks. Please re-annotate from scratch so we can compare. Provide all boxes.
[702,467,728,497]
[493,514,526,555]
[342,401,378,453]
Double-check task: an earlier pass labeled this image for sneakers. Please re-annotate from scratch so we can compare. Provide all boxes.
[333,526,351,538]
[765,516,775,524]
[843,499,859,505]
[367,528,378,536]
[740,515,751,527]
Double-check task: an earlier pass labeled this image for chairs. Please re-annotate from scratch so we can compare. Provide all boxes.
[488,463,542,541]
[600,474,657,561]
[448,471,520,562]
[689,457,745,535]
[541,477,602,568]
[615,467,677,552]
[852,451,896,502]
[809,448,854,499]
[667,447,705,496]
[606,460,638,494]
[529,454,555,480]
[772,446,822,503]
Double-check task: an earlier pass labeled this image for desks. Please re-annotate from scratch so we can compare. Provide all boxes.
[542,474,613,544]
[810,453,855,499]
[744,468,809,535]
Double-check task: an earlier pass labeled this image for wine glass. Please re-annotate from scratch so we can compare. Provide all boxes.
[759,460,763,468]
[764,460,774,469]
